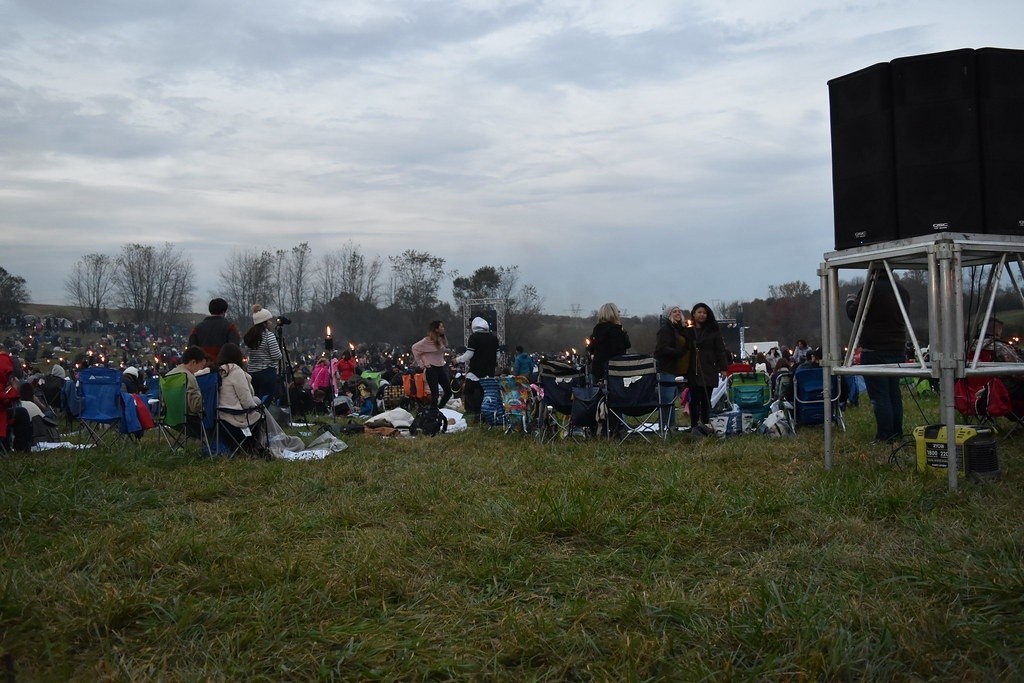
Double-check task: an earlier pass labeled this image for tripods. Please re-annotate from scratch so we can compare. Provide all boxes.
[275,326,309,434]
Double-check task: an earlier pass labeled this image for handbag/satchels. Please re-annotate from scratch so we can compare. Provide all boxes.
[709,409,756,439]
[759,411,795,440]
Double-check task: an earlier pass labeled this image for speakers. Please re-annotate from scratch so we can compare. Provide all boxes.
[823,48,1024,251]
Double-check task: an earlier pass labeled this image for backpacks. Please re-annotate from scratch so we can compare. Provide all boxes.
[570,387,604,428]
[31,415,60,446]
[318,420,367,437]
[409,410,448,437]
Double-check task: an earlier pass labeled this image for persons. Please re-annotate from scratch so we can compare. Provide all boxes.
[845,269,909,445]
[728,317,1024,426]
[186,298,240,364]
[0,313,564,458]
[654,304,688,431]
[586,303,631,439]
[686,303,729,432]
[243,305,282,409]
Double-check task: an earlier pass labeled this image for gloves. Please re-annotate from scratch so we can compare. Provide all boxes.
[846,293,857,301]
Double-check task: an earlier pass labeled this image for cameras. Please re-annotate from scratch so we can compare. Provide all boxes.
[277,316,291,326]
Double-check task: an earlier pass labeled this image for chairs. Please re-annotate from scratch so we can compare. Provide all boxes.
[0,365,296,462]
[498,353,849,449]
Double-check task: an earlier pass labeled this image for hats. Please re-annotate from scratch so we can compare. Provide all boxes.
[661,305,680,319]
[315,359,331,369]
[124,367,138,377]
[252,305,272,325]
[50,365,66,380]
